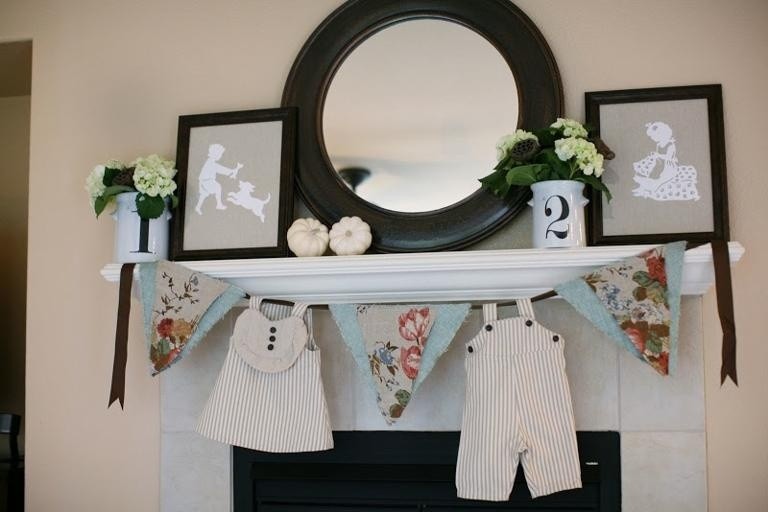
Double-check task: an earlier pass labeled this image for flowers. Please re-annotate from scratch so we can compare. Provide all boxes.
[478,118,616,203]
[85,153,179,221]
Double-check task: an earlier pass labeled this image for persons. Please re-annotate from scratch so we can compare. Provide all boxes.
[194,143,237,217]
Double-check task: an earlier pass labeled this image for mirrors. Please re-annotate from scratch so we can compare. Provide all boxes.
[278,0,566,252]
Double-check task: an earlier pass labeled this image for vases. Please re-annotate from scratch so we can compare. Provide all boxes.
[114,192,168,262]
[529,180,589,248]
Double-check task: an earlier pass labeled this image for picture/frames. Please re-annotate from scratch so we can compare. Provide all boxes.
[584,83,730,246]
[169,108,295,259]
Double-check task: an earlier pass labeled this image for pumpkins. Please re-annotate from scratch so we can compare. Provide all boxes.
[287,217,329,256]
[330,216,373,256]
